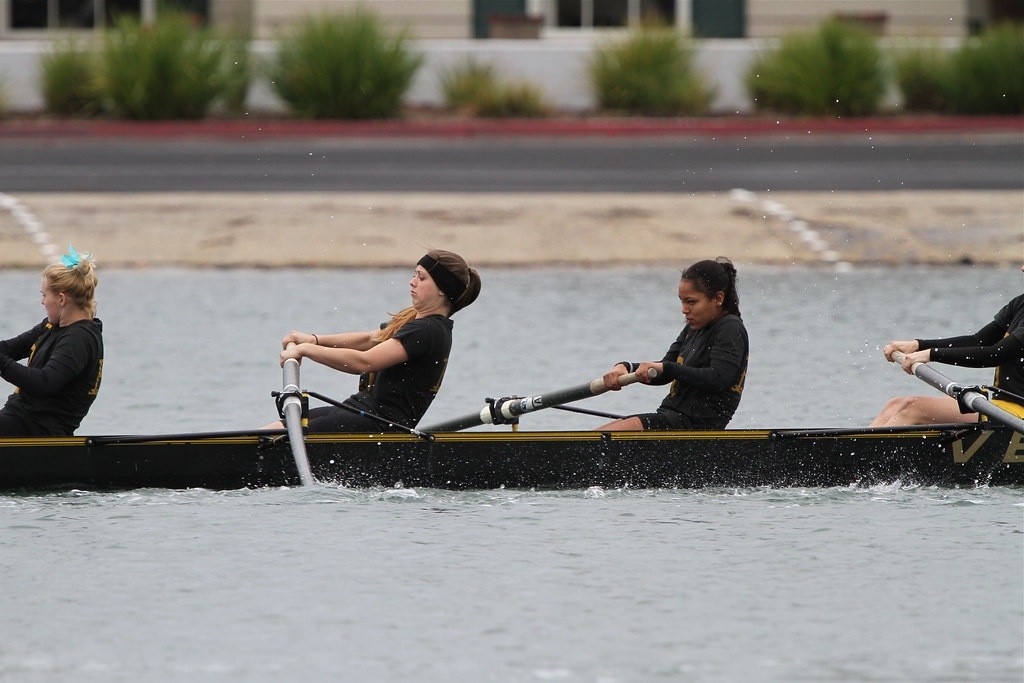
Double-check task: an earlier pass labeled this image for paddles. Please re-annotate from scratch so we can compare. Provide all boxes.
[415,366,657,434]
[276,343,317,486]
[891,351,1024,434]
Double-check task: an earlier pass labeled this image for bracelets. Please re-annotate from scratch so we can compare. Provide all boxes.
[313,334,319,345]
[627,362,634,373]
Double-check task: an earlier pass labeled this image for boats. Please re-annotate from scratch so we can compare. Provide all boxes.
[0,421,1024,495]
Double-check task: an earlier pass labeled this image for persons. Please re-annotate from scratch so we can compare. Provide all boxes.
[255,249,483,433]
[871,265,1024,429]
[598,258,751,433]
[0,253,104,437]
